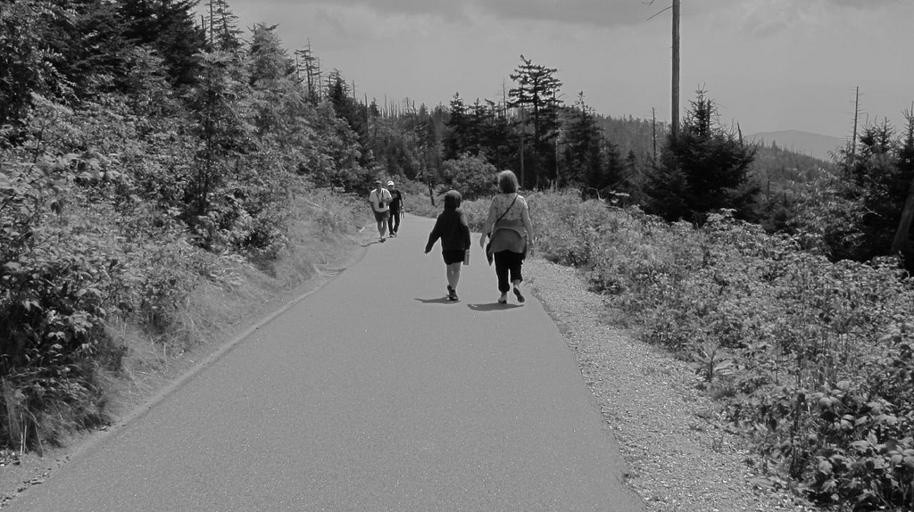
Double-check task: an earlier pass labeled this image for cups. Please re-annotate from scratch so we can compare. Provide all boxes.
[464,252,470,265]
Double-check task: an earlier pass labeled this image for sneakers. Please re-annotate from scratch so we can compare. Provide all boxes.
[447,285,458,301]
[498,285,525,304]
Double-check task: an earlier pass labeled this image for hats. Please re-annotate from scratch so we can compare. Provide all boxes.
[388,181,394,186]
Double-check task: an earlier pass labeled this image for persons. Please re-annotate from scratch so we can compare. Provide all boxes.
[423,190,471,303]
[479,168,535,305]
[382,180,404,238]
[368,181,392,243]
